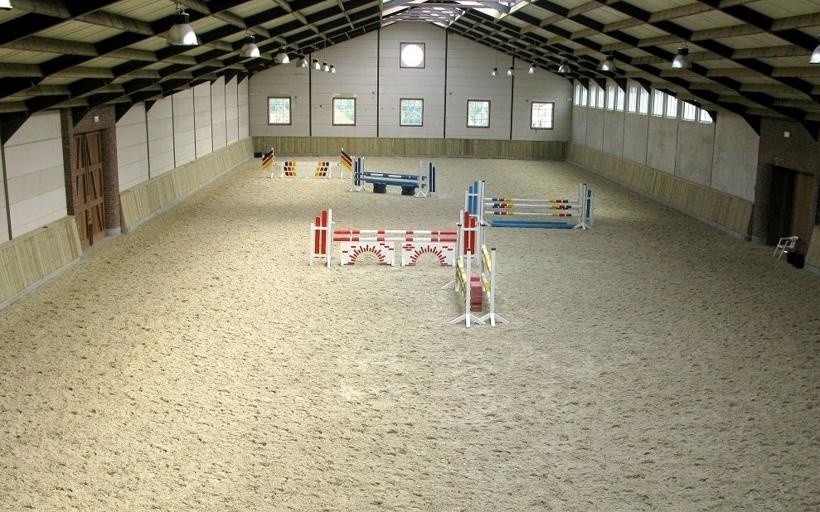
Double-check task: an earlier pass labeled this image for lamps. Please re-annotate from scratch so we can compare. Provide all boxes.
[239,28,261,58]
[671,41,691,69]
[491,40,538,76]
[557,60,571,73]
[167,0,200,48]
[274,43,336,73]
[809,43,820,63]
[600,50,616,71]
